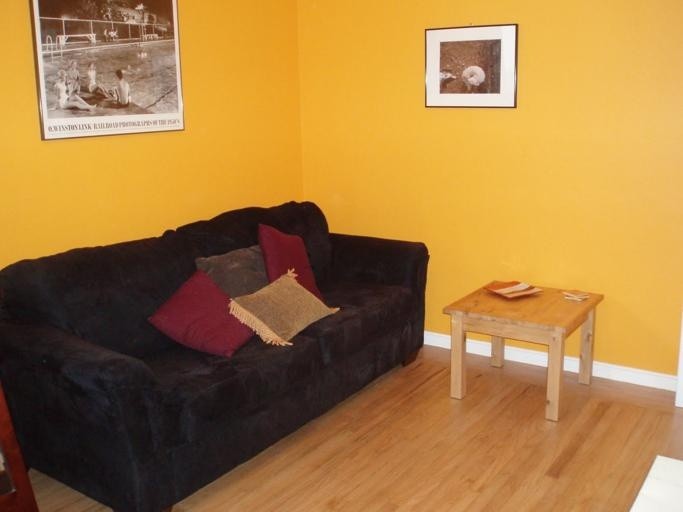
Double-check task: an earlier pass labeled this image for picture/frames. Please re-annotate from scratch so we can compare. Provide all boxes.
[425,24,518,108]
[29,0,185,141]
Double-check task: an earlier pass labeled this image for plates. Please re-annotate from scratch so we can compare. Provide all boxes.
[483,281,542,298]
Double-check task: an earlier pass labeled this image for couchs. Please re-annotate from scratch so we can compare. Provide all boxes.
[0,201,430,512]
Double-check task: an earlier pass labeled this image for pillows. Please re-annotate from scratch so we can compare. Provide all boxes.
[147,223,340,360]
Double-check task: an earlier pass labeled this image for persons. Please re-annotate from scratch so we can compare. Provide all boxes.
[48,60,131,111]
[103,27,118,42]
